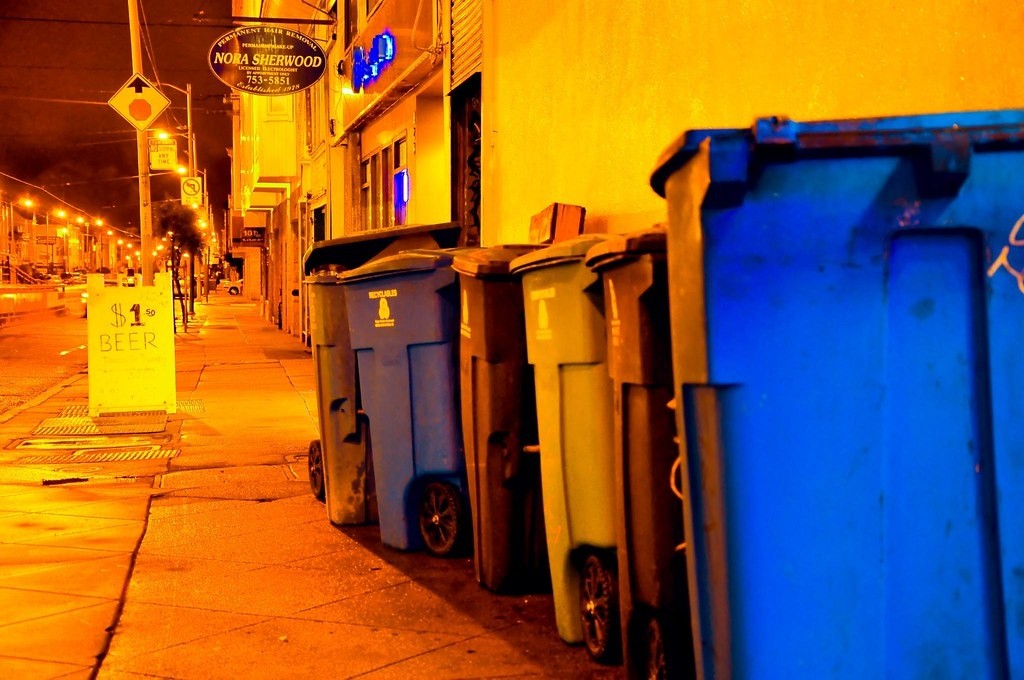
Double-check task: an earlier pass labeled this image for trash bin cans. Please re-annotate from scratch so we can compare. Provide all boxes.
[582,221,694,680]
[334,247,473,557]
[450,244,551,594]
[302,221,463,526]
[648,108,1024,680]
[508,233,622,665]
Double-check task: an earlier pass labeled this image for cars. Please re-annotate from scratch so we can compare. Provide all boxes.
[223,279,243,295]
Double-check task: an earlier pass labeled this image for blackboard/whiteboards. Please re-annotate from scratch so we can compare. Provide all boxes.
[86,273,176,416]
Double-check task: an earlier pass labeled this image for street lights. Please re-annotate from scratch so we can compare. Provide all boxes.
[46,211,64,269]
[10,201,32,241]
[177,165,209,295]
[159,132,202,301]
